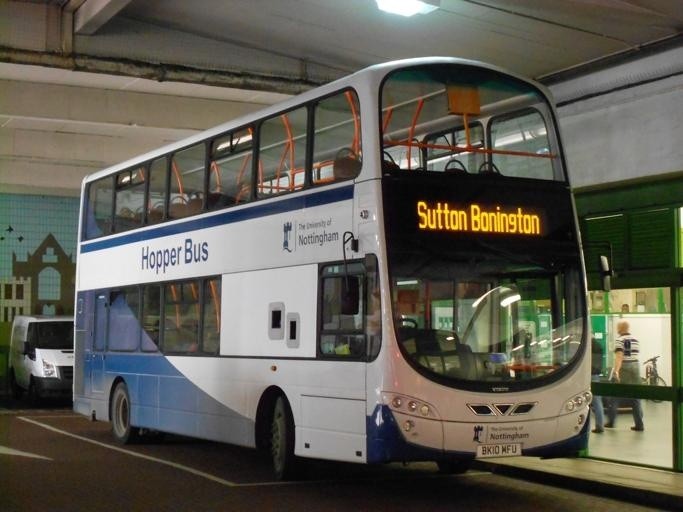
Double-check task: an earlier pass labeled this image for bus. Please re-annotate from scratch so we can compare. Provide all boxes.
[72,56,617,479]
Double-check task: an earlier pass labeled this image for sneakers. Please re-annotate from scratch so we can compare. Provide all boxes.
[591,423,644,434]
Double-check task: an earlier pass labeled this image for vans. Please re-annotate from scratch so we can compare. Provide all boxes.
[8,313,74,407]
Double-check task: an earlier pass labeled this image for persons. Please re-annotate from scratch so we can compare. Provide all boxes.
[604,322,644,431]
[589,333,605,433]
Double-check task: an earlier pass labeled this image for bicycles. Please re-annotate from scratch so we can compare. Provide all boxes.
[642,355,666,403]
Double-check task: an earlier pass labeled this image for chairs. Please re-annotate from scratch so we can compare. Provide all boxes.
[383,149,400,172]
[444,159,469,174]
[102,192,203,234]
[332,145,361,181]
[478,161,503,175]
